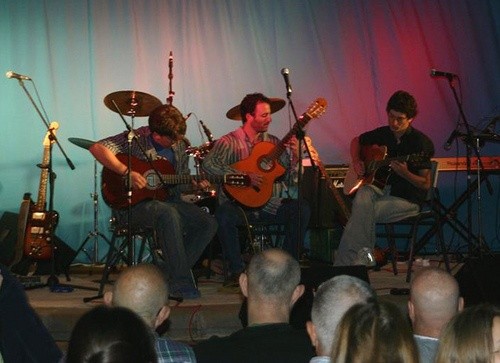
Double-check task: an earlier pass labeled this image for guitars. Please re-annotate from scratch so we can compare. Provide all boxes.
[344,144,416,195]
[101,154,250,210]
[223,98,328,210]
[25,119,59,264]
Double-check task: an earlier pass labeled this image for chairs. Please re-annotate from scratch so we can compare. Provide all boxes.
[99,207,159,296]
[374,159,451,282]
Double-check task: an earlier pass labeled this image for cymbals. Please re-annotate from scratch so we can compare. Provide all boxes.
[102,90,163,117]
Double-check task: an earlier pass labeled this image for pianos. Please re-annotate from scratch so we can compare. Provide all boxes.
[414,155,500,257]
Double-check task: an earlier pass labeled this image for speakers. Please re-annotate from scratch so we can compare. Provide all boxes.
[237,265,371,328]
[0,199,77,277]
[300,164,357,264]
[454,260,500,310]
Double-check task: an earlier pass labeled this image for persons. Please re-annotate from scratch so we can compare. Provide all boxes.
[334,90,435,266]
[436,303,500,363]
[306,275,375,363]
[202,92,310,287]
[330,301,419,363]
[67,305,155,363]
[90,104,219,297]
[407,268,464,363]
[0,274,66,363]
[104,263,197,363]
[197,248,314,363]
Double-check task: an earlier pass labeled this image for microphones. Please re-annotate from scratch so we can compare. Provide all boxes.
[183,113,192,121]
[168,51,173,79]
[281,67,292,95]
[162,184,171,194]
[199,120,213,141]
[430,69,457,78]
[6,71,31,80]
[443,129,458,150]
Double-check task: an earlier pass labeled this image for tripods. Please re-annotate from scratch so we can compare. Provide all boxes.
[75,161,129,277]
